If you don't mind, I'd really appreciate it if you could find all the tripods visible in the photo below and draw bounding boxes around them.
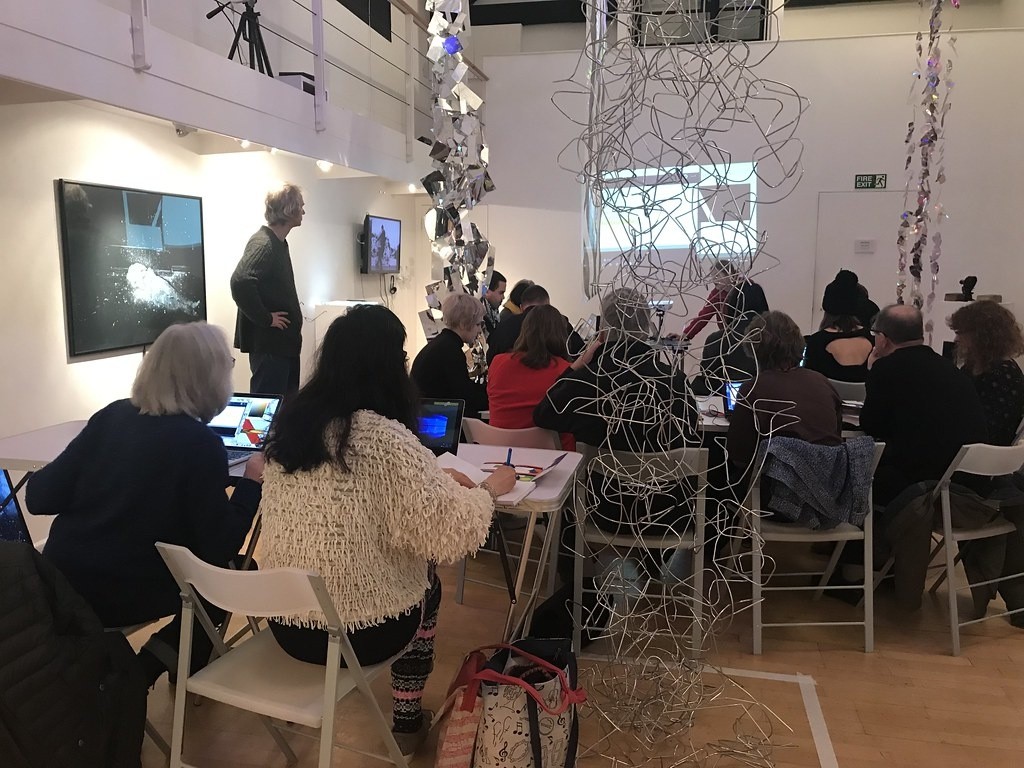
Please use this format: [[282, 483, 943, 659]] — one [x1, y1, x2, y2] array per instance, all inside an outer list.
[[206, 0, 275, 78]]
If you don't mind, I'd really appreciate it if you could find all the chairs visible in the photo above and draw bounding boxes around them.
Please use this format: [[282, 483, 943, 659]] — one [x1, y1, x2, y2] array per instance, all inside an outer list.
[[154, 540, 429, 768], [455, 416, 561, 605], [827, 376, 867, 402], [572, 443, 708, 663], [0, 541, 174, 768], [722, 438, 886, 656], [857, 438, 1024, 658]]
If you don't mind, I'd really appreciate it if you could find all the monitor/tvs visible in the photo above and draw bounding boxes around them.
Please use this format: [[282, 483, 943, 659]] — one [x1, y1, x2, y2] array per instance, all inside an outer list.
[[360, 215, 401, 274]]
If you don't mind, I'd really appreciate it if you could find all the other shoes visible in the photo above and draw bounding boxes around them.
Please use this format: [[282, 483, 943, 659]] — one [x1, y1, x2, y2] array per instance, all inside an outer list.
[[558, 554, 576, 588], [812, 572, 863, 607], [169, 640, 211, 686], [596, 554, 640, 613], [661, 547, 693, 598]]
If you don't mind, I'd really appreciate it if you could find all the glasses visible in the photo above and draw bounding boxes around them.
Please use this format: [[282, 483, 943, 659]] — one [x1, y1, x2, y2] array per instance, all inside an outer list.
[[472, 319, 485, 330], [870, 323, 889, 339]]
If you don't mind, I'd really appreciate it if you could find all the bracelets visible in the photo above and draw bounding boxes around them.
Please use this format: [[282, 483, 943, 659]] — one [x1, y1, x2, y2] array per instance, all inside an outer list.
[[479, 481, 496, 501]]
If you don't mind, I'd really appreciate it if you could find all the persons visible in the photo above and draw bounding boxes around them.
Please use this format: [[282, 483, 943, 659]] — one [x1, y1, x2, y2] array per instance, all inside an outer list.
[[230, 183, 305, 410], [26, 320, 266, 685], [375, 224, 386, 270], [258, 304, 518, 765], [410, 260, 1024, 608]]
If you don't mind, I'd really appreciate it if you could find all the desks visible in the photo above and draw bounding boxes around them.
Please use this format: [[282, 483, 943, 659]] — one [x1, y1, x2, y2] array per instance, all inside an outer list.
[[696, 396, 863, 440], [0, 420, 583, 644]]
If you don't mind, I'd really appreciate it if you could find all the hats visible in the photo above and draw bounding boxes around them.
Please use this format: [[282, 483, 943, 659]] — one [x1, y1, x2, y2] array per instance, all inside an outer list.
[[823, 269, 869, 315], [601, 288, 650, 331]]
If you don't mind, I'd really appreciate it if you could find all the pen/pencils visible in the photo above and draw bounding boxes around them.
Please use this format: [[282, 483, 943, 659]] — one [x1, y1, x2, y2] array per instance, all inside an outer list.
[[506, 448, 512, 466]]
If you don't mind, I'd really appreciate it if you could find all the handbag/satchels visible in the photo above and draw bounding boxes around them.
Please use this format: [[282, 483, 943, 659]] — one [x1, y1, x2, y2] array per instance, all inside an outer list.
[[433, 636, 590, 768], [529, 575, 613, 650]]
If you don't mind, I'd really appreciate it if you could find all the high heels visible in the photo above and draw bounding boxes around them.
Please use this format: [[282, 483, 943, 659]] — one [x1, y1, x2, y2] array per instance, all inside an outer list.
[[393, 707, 434, 756]]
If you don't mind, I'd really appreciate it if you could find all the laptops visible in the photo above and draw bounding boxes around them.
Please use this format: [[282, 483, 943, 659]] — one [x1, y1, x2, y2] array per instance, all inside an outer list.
[[205, 392, 284, 467], [722, 378, 744, 419], [413, 398, 465, 457]]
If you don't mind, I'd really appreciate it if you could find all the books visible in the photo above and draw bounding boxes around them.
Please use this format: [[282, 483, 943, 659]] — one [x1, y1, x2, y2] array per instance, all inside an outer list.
[[435, 452, 537, 508]]
